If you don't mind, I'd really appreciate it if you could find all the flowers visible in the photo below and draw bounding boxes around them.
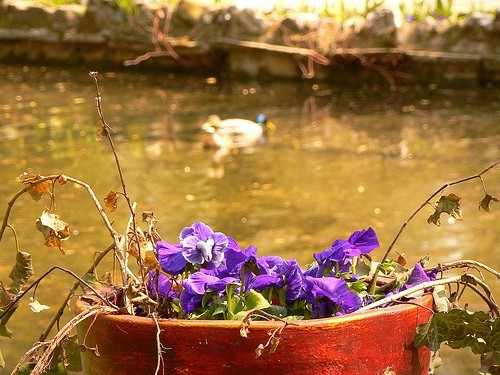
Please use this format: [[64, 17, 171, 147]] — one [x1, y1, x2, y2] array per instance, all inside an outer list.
[[0, 71, 500, 375]]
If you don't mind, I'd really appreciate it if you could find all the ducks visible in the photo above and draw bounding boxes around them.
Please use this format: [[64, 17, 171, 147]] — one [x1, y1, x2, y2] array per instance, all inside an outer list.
[[202, 112, 277, 145]]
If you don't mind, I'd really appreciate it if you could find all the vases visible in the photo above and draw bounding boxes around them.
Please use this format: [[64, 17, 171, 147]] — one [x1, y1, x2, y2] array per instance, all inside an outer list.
[[75, 273, 441, 375]]
